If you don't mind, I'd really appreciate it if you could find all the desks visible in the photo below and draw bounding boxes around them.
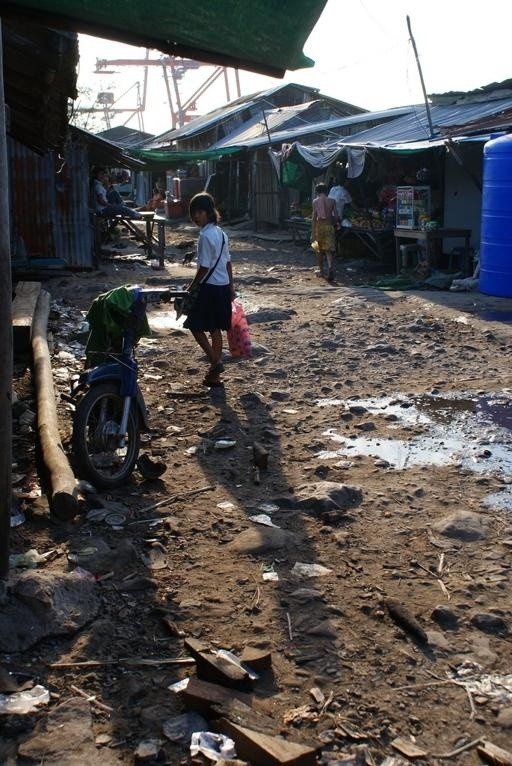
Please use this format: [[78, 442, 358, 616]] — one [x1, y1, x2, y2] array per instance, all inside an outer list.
[[92, 213, 167, 270], [285, 212, 392, 271], [393, 227, 474, 279]]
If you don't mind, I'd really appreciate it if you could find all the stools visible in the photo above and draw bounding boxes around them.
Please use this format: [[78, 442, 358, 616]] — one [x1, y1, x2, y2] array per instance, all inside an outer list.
[[398, 241, 426, 272], [444, 240, 479, 276]]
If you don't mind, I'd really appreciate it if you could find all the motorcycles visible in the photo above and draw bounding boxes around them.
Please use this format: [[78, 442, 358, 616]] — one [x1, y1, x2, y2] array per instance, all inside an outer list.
[[72, 282, 189, 492]]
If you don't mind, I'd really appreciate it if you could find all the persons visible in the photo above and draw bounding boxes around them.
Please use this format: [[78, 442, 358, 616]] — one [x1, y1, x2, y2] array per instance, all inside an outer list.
[[327, 179, 366, 243], [309, 183, 341, 280], [177, 192, 235, 388], [93, 171, 144, 221], [155, 177, 164, 195], [144, 188, 164, 206]]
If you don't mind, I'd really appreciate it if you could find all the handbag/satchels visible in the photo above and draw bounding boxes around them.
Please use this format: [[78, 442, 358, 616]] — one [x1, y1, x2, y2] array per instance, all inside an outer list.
[[174, 283, 199, 313]]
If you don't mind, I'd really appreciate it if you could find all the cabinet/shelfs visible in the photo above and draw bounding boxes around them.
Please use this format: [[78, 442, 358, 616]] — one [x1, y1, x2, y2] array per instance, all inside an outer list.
[[396, 184, 431, 229]]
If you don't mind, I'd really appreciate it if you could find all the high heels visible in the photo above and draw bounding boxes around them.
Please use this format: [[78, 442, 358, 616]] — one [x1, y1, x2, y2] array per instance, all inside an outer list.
[[204, 360, 224, 384]]
